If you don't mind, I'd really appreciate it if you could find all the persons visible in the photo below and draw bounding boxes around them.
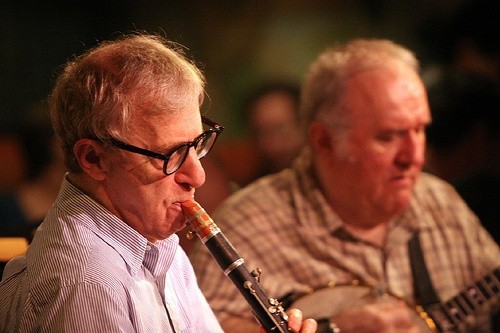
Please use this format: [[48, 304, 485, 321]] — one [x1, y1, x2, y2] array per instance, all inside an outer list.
[[240, 74, 308, 177], [0, 34, 320, 332], [186, 36, 500, 333], [0, 94, 69, 283]]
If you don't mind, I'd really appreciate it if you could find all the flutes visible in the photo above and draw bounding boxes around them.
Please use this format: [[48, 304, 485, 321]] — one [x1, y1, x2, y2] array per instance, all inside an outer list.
[[182, 198, 296, 333]]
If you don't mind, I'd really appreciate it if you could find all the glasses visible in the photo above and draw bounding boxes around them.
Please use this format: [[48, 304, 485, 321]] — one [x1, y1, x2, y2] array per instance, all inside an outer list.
[[88, 116, 225, 175]]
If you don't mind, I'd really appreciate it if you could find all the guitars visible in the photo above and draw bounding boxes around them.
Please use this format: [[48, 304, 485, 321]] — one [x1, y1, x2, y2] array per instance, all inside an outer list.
[[279, 264, 500, 333]]
